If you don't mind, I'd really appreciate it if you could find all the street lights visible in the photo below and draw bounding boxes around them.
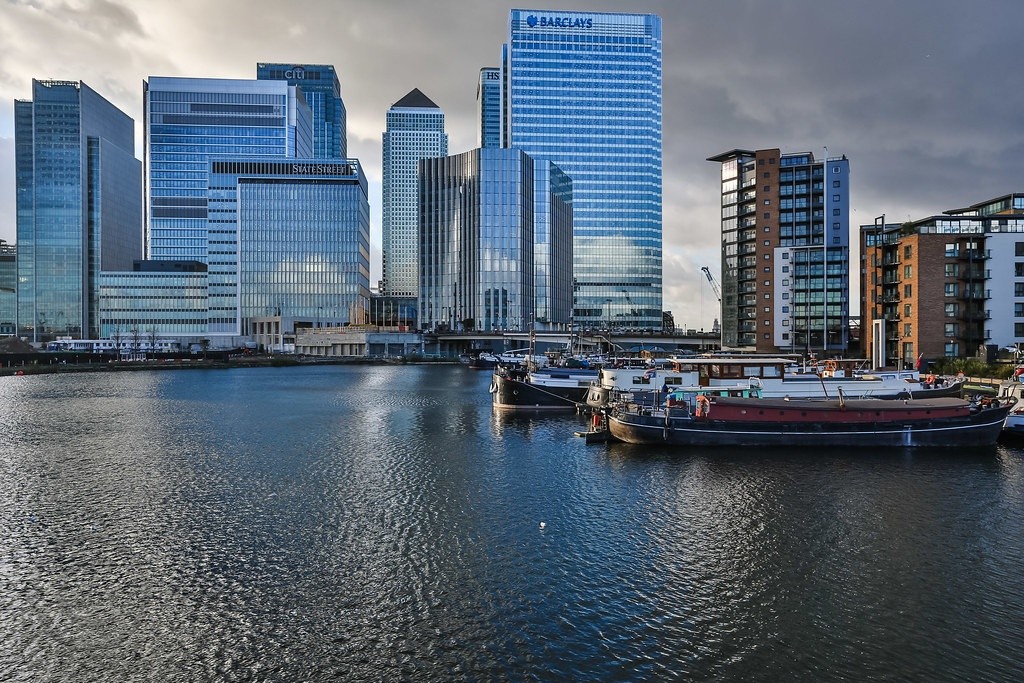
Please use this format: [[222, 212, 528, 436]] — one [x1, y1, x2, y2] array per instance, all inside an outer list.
[[606, 299, 614, 361]]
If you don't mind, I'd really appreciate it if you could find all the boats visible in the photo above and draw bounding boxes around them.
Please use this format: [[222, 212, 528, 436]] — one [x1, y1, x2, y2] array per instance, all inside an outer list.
[[466, 310, 1018, 452], [993, 368, 1024, 441]]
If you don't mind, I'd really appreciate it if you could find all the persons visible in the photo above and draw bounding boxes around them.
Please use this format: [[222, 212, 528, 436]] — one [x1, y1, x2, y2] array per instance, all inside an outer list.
[[895, 388, 914, 400]]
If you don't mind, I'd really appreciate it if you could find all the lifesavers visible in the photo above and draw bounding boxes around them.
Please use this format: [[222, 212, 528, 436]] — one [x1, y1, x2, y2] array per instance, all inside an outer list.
[[651, 359, 655, 363], [927, 374, 934, 384]]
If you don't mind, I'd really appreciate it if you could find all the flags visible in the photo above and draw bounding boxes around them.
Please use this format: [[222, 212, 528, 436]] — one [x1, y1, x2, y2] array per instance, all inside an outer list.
[[644, 368, 656, 379]]
